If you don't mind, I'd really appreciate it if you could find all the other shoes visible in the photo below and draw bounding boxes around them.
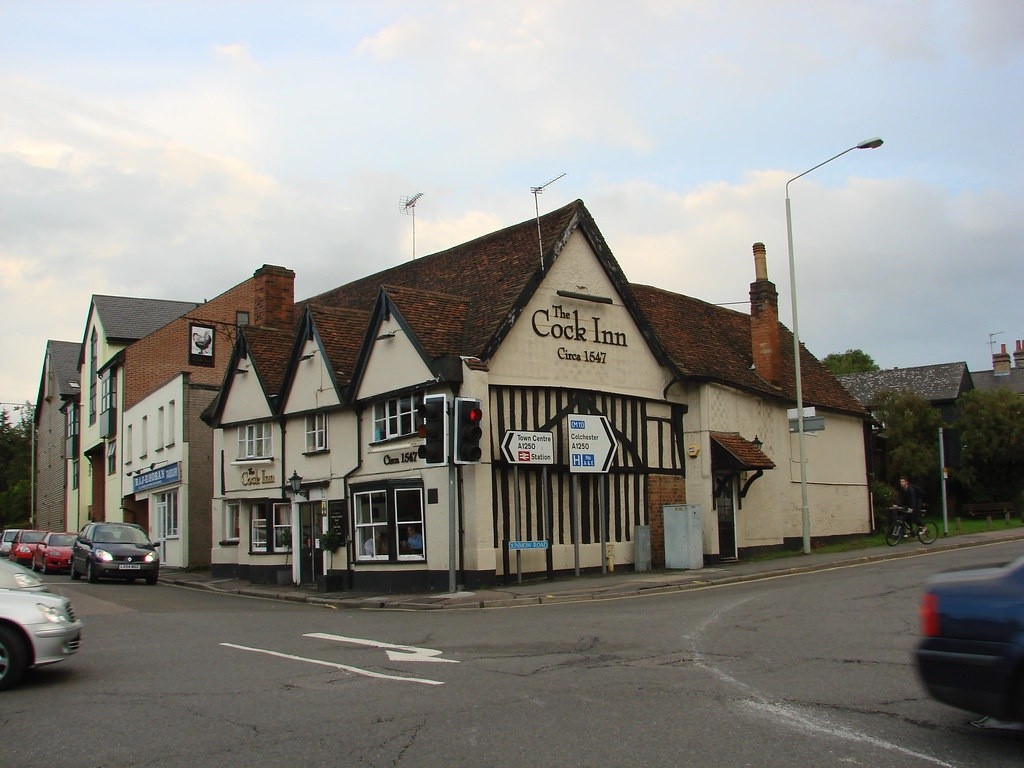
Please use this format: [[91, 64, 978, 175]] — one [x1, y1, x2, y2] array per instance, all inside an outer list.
[[918, 527, 928, 535], [903, 533, 911, 537]]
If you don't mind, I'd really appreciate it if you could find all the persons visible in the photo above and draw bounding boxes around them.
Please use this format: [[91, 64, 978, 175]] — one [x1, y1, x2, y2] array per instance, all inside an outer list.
[[406, 525, 422, 549], [893, 476, 928, 537], [364, 530, 386, 555]]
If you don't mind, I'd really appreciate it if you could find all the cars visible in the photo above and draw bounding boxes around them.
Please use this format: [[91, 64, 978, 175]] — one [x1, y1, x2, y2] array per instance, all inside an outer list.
[[0, 529, 20, 556], [9, 530, 49, 564], [32, 531, 81, 575], [909, 552, 1023, 725], [70, 522, 161, 585], [0, 588, 83, 692], [0, 556, 50, 593]]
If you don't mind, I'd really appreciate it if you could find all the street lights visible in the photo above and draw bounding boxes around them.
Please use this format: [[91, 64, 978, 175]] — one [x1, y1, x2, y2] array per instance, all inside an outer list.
[[785, 134, 885, 556]]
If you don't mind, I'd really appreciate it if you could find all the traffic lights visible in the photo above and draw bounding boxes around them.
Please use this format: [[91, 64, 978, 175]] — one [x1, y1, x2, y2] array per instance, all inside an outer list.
[[416, 393, 446, 465], [451, 397, 483, 465]]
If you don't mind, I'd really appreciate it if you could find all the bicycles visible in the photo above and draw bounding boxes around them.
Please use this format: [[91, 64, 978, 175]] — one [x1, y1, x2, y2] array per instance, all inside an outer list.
[[886, 503, 939, 547]]
